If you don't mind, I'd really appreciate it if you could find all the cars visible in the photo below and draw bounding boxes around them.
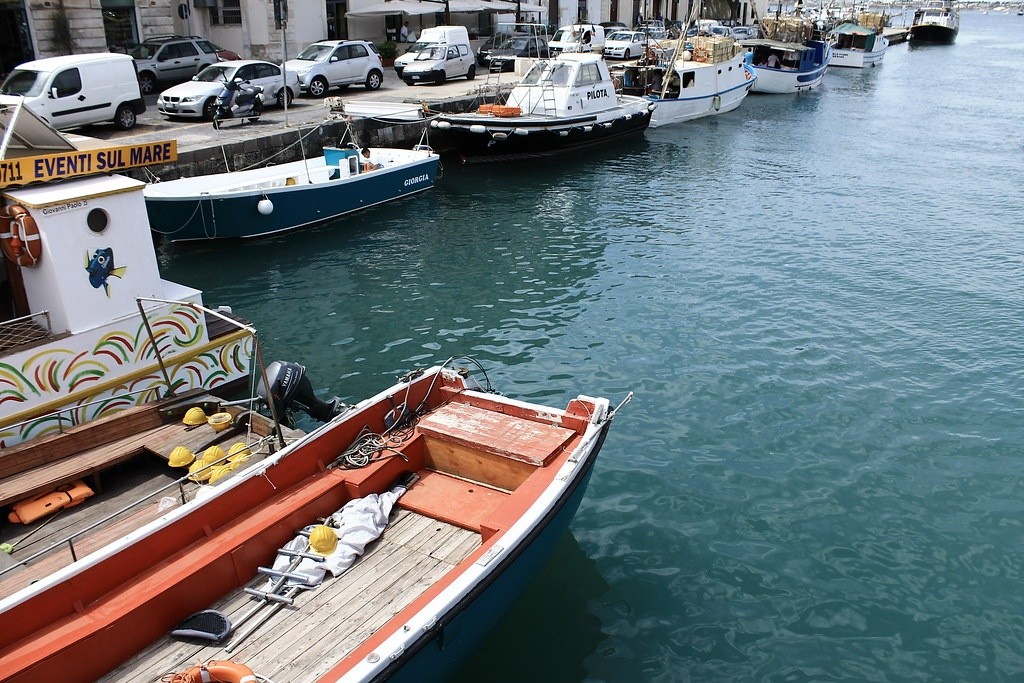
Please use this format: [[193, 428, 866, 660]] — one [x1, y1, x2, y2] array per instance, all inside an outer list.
[[156, 59, 300, 122], [476, 31, 534, 69], [484, 36, 549, 72], [573, 15, 759, 59], [211, 43, 242, 62], [401, 43, 477, 86]]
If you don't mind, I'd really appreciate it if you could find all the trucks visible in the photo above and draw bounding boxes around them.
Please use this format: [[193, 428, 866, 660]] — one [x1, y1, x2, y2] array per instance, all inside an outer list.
[[393, 26, 470, 80]]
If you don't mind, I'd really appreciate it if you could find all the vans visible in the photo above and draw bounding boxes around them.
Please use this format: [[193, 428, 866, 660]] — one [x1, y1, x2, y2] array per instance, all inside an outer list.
[[548, 24, 606, 54], [0, 53, 146, 134]]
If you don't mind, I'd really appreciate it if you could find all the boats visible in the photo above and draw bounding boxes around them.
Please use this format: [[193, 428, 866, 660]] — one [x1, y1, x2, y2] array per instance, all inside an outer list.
[[422, 51, 659, 168], [142, 95, 440, 249], [908, 0, 960, 41], [981, 2, 1024, 16], [740, 0, 834, 95], [0, 355, 614, 683], [825, 21, 890, 68], [0, 178, 353, 596], [771, 0, 902, 41], [609, 0, 759, 129]]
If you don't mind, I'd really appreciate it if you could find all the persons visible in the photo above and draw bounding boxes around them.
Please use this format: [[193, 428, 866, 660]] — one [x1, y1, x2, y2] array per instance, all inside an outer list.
[[361, 148, 381, 172], [647, 70, 662, 95], [614, 76, 621, 94], [624, 68, 634, 87], [519, 16, 526, 32], [530, 17, 537, 28], [744, 47, 754, 65], [401, 21, 409, 43], [767, 53, 780, 68]]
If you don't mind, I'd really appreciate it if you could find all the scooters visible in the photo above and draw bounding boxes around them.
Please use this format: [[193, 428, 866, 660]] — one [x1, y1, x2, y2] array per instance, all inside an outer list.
[[211, 68, 264, 129]]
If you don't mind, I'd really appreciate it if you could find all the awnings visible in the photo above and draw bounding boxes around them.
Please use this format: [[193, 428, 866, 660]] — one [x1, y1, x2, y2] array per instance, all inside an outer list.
[[344, 0, 547, 18]]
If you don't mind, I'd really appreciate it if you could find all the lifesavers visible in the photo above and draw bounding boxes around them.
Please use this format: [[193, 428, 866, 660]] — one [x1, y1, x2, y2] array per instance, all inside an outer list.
[[693, 46, 708, 62], [168, 659, 261, 683], [479, 103, 497, 114], [491, 105, 520, 117], [0, 204, 41, 268]]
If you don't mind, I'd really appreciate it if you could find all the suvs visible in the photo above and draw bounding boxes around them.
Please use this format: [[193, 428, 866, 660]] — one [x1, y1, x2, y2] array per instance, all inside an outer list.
[[280, 38, 384, 99], [124, 35, 220, 97]]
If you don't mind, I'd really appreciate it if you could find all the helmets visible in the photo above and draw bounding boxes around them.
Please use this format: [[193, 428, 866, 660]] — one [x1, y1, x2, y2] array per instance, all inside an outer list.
[[226, 443, 252, 461], [230, 454, 249, 472], [169, 445, 195, 466], [256, 92, 266, 105], [206, 412, 232, 431], [188, 459, 213, 480], [202, 445, 225, 469], [210, 467, 232, 485], [309, 525, 337, 556], [182, 406, 209, 425]]
